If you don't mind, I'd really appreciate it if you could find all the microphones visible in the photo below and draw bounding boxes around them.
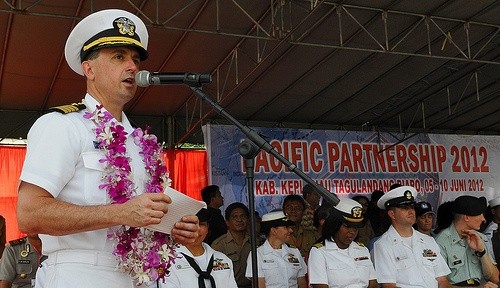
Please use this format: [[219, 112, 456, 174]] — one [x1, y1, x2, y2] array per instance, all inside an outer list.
[[135, 70, 212, 87]]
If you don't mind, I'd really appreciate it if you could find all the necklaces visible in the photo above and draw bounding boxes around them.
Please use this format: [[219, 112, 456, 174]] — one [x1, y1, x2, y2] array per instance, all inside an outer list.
[[80, 101, 184, 288]]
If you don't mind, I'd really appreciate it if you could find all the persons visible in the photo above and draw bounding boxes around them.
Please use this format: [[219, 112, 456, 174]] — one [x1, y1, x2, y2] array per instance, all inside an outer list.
[[245, 211, 308, 288], [16, 9, 200, 288], [370, 186, 453, 288], [248, 184, 500, 269], [211, 202, 263, 288], [160, 207, 238, 288], [435, 197, 500, 288], [307, 198, 377, 288], [196, 184, 228, 246], [0, 233, 43, 288]]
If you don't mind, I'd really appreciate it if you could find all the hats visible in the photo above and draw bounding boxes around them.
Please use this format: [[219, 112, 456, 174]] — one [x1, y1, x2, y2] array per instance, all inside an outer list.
[[262, 211, 296, 227], [488, 197, 500, 207], [452, 195, 485, 215], [65, 9, 149, 76], [415, 201, 436, 217], [195, 201, 215, 222], [328, 198, 367, 227], [377, 186, 418, 210]]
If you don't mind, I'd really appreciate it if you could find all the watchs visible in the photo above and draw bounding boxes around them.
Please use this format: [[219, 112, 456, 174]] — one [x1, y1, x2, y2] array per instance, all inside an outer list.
[[474, 249, 486, 258]]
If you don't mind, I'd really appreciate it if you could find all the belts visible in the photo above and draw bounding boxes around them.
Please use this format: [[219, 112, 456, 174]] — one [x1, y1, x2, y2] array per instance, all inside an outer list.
[[454, 279, 480, 287]]
